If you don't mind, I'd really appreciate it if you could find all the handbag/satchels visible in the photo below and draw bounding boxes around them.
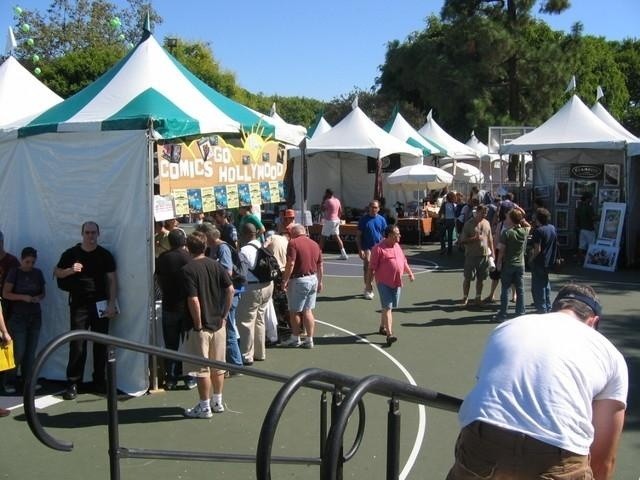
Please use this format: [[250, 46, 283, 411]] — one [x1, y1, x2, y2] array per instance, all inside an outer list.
[[0, 339, 16, 371], [177, 308, 193, 331], [56, 258, 72, 291], [216, 243, 242, 280], [481, 246, 488, 256]]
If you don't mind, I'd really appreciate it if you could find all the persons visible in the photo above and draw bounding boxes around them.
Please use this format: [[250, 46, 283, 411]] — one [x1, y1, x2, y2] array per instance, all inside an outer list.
[[377, 196, 398, 236], [51, 222, 117, 399], [366, 225, 415, 345], [424, 188, 557, 322], [447, 283, 629, 478], [354, 200, 387, 300], [3, 247, 46, 396], [0, 232, 21, 395], [158, 203, 322, 420], [318, 189, 349, 260], [0, 301, 12, 418]]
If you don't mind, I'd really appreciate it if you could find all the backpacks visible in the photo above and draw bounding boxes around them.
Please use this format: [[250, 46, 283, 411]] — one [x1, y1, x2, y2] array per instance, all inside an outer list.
[[245, 243, 281, 282]]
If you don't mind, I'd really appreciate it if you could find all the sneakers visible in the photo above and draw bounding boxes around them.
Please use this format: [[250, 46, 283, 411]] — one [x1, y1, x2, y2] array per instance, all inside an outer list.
[[0, 409, 10, 417], [364, 291, 374, 300], [336, 256, 348, 260], [531, 303, 550, 313], [185, 401, 224, 418], [463, 297, 517, 323], [265, 339, 314, 348], [166, 379, 197, 390], [380, 327, 397, 344], [440, 247, 446, 255]]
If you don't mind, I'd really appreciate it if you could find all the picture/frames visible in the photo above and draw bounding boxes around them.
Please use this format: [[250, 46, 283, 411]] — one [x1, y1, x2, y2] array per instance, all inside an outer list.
[[533, 163, 627, 271]]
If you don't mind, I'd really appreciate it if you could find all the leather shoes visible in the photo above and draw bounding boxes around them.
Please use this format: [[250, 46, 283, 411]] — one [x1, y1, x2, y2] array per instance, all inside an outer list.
[[63, 384, 77, 399], [94, 384, 108, 393]]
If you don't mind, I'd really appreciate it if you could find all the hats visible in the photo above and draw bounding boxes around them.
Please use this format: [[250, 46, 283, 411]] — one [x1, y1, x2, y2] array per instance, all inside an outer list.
[[284, 209, 295, 217]]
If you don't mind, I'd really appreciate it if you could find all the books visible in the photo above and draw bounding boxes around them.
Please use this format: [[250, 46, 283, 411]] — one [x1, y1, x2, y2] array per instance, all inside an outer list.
[[96, 299, 120, 318]]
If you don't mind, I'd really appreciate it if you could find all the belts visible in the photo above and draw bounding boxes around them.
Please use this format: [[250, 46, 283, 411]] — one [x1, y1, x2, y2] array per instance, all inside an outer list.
[[291, 272, 314, 278], [248, 281, 261, 284]]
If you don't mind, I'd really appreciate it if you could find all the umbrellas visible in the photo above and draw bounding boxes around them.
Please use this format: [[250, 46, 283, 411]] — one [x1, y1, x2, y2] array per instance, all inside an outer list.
[[387, 163, 453, 246], [440, 162, 484, 191]]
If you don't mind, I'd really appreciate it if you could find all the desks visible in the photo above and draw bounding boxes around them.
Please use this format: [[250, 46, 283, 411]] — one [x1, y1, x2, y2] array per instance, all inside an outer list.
[[153, 301, 167, 387], [308, 206, 444, 250]]
